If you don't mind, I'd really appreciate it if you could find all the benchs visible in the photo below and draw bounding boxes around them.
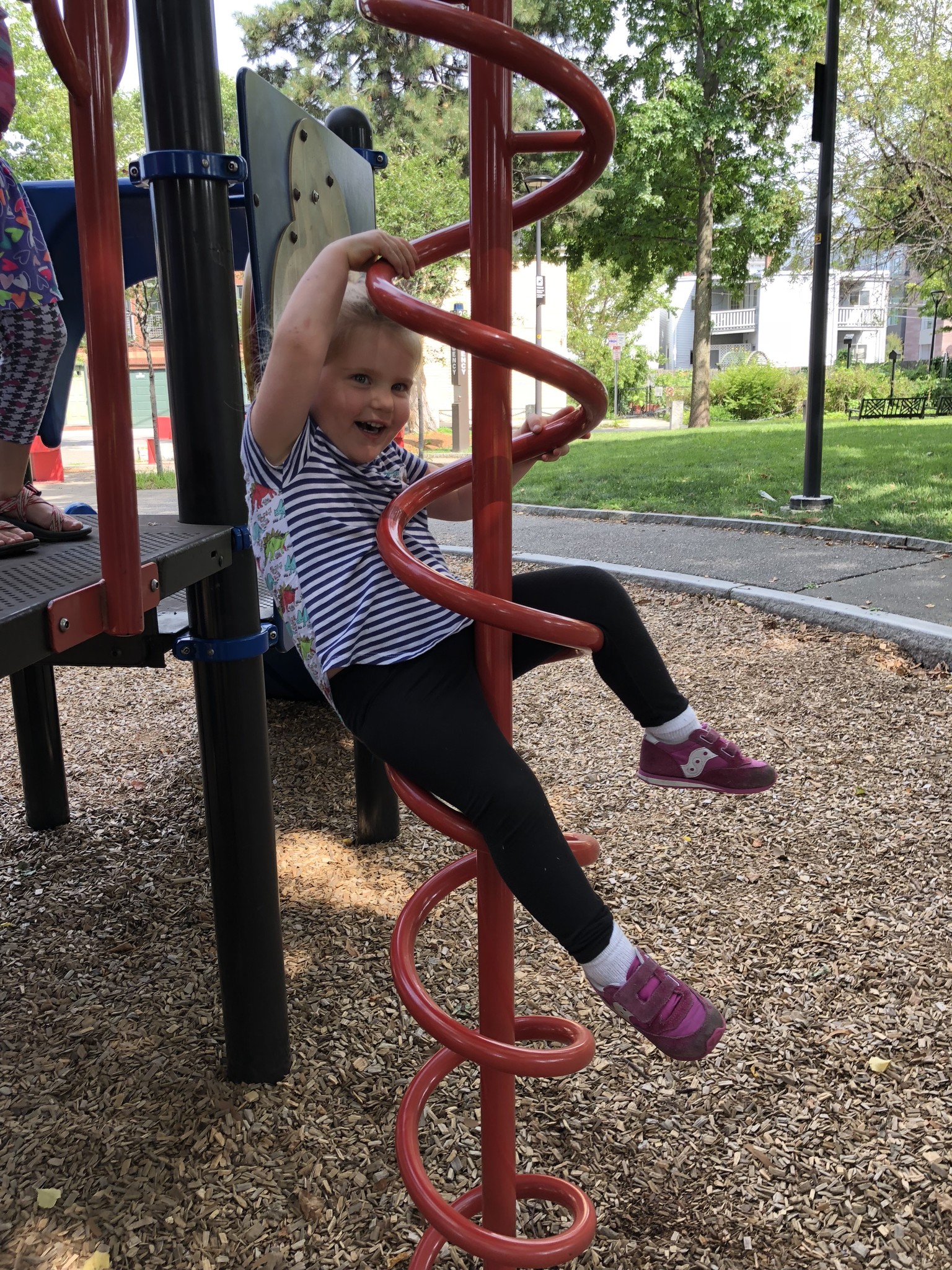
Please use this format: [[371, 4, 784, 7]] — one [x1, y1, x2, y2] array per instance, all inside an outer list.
[[922, 396, 952, 418], [848, 397, 927, 422]]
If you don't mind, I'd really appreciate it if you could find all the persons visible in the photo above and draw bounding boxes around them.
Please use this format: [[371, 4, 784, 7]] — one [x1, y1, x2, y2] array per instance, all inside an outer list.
[[238, 232, 778, 1065], [0, 13, 92, 561]]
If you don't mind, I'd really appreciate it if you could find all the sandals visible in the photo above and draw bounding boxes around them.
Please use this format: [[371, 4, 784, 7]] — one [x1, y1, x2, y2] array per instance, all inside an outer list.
[[0, 486, 93, 541], [0, 521, 40, 555]]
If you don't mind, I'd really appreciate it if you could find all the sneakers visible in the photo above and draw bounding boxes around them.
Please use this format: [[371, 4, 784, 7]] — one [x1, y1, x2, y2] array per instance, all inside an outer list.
[[585, 946, 726, 1062], [637, 723, 777, 794]]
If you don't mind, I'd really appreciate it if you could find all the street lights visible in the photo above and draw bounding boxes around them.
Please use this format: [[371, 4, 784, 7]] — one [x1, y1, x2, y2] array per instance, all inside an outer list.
[[927, 290, 946, 376], [523, 174, 556, 416]]
